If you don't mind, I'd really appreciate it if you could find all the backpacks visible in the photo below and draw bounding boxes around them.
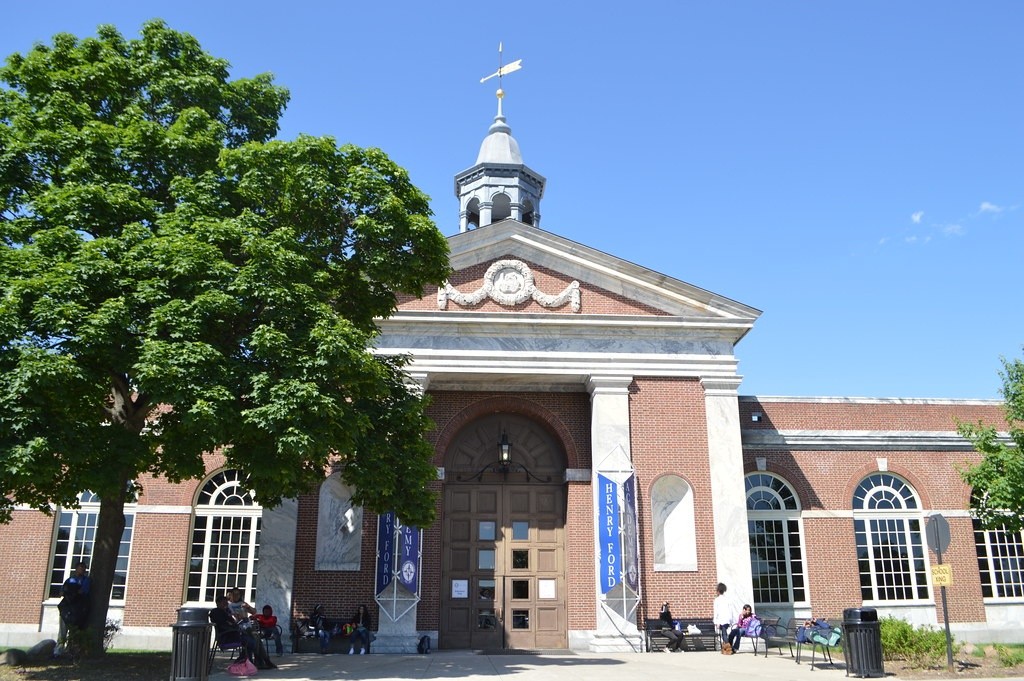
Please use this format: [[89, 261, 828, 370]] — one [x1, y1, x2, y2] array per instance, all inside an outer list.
[[744, 618, 762, 637]]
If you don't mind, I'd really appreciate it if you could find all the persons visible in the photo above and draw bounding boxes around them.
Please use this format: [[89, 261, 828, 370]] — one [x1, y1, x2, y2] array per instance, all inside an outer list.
[[210, 596, 256, 664], [249, 605, 283, 657], [660, 602, 685, 654], [728, 604, 757, 653], [713, 583, 734, 655], [348, 605, 371, 655], [305, 604, 331, 654], [226, 588, 277, 670], [53, 562, 91, 659], [798, 617, 842, 647]]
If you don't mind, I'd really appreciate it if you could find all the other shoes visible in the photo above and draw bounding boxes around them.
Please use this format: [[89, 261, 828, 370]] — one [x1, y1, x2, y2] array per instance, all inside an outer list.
[[829, 628, 841, 646], [277, 653, 282, 657], [360, 648, 365, 655], [349, 648, 354, 654], [54, 641, 63, 657], [675, 648, 684, 653], [322, 649, 328, 653], [663, 647, 670, 653], [722, 643, 733, 654], [810, 631, 828, 645], [264, 658, 277, 669], [257, 661, 270, 670]]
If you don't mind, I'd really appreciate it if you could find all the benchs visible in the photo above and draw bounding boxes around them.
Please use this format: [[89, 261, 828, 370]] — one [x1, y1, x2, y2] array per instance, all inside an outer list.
[[208, 614, 283, 674], [731, 616, 782, 656], [643, 617, 722, 652], [797, 618, 850, 677], [765, 618, 825, 662], [290, 618, 370, 654]]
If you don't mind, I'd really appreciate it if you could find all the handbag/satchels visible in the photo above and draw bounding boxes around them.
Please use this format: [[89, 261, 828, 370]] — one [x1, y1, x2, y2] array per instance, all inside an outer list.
[[228, 657, 257, 675], [687, 625, 701, 635], [673, 620, 680, 632]]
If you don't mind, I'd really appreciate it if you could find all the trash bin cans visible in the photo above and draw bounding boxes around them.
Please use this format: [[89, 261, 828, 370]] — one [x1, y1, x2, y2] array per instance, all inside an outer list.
[[841, 607, 886, 678], [169, 608, 216, 681]]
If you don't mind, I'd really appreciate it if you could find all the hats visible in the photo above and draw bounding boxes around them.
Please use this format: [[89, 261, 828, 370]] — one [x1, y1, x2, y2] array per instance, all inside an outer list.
[[216, 595, 226, 603], [314, 604, 324, 611]]
